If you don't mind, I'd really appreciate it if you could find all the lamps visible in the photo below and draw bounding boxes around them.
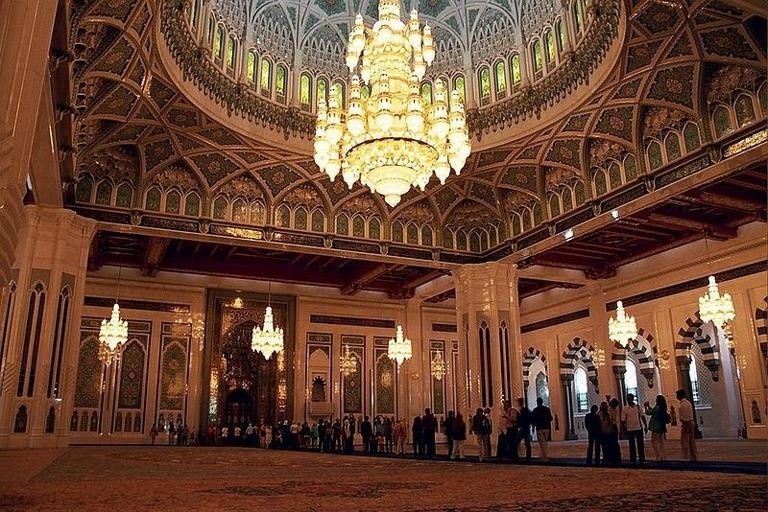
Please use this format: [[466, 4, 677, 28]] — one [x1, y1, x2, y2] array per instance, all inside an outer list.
[[251, 253, 285, 362], [608, 237, 638, 349], [689, 204, 736, 330], [98, 236, 129, 352], [388, 281, 413, 365], [312, 0, 473, 208]]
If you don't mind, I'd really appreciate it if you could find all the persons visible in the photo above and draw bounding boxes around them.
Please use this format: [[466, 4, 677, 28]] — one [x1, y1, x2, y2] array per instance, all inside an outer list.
[[413, 399, 554, 464], [621, 393, 648, 462], [676, 389, 698, 461], [643, 395, 666, 462], [169, 423, 216, 446], [245, 423, 273, 448], [585, 398, 622, 465], [149, 424, 159, 445], [276, 415, 354, 455], [222, 423, 241, 446], [360, 415, 410, 455]]
